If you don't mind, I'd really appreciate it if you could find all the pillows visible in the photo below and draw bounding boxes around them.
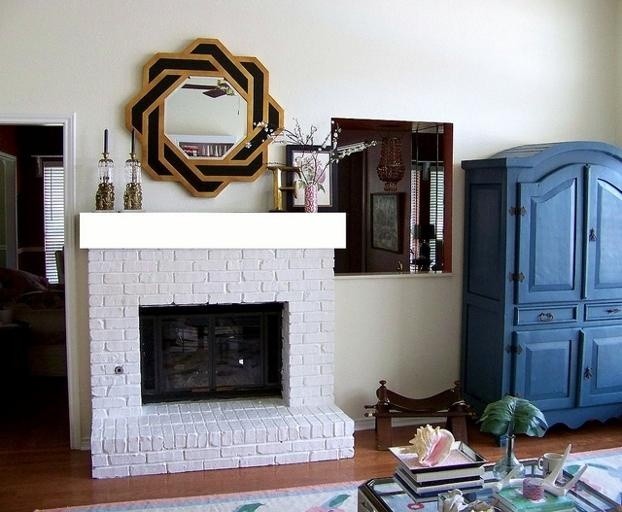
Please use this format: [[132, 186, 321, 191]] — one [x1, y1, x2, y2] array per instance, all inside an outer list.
[[1, 265, 49, 301]]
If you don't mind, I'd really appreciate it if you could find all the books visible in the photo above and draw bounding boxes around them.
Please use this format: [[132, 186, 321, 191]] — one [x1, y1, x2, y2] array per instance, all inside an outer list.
[[392, 467, 576, 512]]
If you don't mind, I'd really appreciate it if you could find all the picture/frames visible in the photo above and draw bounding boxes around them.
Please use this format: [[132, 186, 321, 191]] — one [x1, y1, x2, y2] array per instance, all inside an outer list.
[[369, 192, 402, 253], [287, 145, 337, 213]]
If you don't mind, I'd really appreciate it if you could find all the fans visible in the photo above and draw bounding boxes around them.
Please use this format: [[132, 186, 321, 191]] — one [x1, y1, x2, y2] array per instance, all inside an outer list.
[[182, 78, 242, 115]]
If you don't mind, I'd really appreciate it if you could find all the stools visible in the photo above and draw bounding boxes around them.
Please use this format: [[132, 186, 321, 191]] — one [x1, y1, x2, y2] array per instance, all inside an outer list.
[[365, 381, 474, 442]]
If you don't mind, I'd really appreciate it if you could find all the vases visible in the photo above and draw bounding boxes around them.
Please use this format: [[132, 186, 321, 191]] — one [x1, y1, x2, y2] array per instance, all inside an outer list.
[[304, 184, 318, 213]]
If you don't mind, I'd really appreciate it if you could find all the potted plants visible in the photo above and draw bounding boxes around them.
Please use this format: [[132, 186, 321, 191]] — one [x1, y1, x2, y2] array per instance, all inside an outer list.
[[475, 393, 548, 478]]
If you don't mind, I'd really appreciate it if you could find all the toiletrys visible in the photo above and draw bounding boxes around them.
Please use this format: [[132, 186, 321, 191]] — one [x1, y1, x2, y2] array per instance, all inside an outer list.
[[198, 145, 226, 157]]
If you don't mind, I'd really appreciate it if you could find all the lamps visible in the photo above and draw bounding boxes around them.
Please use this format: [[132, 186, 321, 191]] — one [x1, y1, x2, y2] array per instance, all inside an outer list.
[[414, 225, 433, 259]]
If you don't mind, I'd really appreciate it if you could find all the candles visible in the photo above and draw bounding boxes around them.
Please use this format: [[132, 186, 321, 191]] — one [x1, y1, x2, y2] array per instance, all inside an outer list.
[[132, 126, 136, 153], [103, 129, 109, 152]]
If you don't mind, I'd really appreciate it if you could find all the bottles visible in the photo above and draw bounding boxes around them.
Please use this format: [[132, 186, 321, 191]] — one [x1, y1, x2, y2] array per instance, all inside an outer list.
[[94, 152, 117, 213], [122, 152, 144, 210], [492, 435, 526, 481]]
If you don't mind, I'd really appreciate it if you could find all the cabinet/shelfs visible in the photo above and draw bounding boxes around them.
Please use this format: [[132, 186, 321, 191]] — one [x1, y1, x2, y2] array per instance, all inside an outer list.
[[460, 141, 621, 428]]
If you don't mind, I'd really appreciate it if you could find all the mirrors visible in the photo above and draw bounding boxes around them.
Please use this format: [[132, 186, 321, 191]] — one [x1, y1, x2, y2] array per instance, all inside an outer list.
[[126, 38, 286, 199]]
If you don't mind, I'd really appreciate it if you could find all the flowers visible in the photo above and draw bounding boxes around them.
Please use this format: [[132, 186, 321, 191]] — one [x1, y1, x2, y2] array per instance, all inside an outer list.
[[245, 113, 378, 191]]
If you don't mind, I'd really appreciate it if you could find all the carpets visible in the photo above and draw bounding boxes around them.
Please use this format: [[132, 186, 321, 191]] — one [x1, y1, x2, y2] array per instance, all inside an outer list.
[[34, 446, 621, 512]]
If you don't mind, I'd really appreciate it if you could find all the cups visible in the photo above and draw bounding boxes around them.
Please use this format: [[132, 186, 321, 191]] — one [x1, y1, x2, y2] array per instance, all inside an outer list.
[[537, 452, 564, 480]]
